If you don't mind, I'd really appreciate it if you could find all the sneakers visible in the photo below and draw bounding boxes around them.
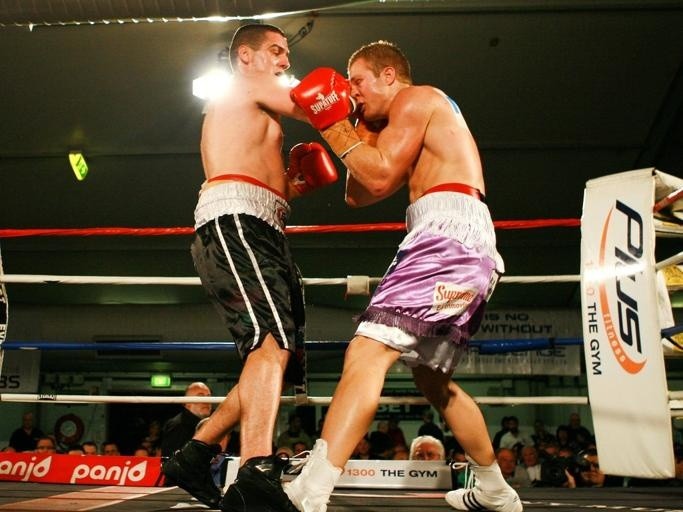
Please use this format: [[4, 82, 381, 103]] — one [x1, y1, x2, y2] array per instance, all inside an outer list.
[[161, 436, 222, 510], [221, 452, 303, 511], [443, 453, 523, 512]]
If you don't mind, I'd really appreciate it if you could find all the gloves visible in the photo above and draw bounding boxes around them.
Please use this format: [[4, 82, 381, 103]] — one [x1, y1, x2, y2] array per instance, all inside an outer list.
[[283, 139, 338, 198], [288, 65, 352, 133]]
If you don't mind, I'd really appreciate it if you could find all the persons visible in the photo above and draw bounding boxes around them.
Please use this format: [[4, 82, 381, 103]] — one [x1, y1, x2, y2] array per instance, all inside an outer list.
[[346, 408, 683, 490], [0, 409, 164, 458], [279, 39, 523, 512], [161, 23, 359, 512], [161, 380, 331, 485]]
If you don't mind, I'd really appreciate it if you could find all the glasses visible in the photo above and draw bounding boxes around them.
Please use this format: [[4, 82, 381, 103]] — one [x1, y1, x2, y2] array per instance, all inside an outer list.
[[35, 445, 56, 450]]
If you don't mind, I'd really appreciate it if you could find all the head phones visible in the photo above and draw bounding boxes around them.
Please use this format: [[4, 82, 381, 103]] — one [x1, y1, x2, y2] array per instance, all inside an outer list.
[[574, 448, 596, 479]]
